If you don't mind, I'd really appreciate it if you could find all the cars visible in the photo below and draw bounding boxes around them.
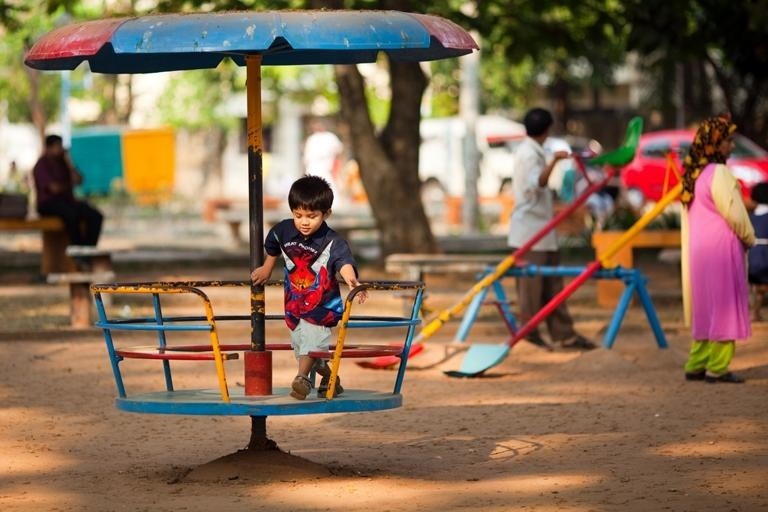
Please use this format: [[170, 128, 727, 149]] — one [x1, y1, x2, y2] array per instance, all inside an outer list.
[[415, 112, 547, 219], [619, 123, 768, 217]]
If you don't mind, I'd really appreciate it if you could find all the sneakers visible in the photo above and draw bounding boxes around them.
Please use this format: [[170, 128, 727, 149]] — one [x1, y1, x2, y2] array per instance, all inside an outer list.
[[290, 375, 312, 400], [317, 377, 343, 398], [525, 335, 595, 349]]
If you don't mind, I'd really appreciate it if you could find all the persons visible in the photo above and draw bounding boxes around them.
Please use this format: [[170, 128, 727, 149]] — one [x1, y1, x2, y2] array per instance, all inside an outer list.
[[6, 161, 21, 189], [33, 135, 103, 271], [302, 121, 345, 215], [506, 108, 597, 352], [748, 178, 768, 285], [649, 107, 665, 132], [683, 111, 757, 383], [250, 175, 369, 400]]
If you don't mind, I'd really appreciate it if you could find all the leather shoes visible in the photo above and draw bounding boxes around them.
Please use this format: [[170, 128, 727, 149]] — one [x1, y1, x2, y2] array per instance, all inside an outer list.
[[686, 371, 744, 382]]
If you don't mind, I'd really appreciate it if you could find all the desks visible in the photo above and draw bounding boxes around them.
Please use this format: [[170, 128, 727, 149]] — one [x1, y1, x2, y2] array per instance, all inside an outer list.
[[0, 216, 74, 276]]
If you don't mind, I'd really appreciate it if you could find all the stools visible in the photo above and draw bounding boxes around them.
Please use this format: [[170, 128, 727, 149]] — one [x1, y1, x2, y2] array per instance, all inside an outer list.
[[45, 241, 131, 331]]
